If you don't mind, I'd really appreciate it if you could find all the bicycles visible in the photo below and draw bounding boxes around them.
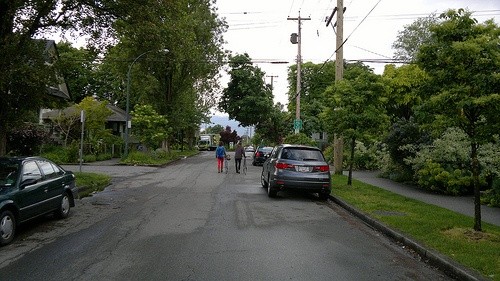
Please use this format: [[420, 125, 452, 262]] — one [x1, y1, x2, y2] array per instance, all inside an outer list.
[[242, 155, 250, 174], [224, 154, 231, 175]]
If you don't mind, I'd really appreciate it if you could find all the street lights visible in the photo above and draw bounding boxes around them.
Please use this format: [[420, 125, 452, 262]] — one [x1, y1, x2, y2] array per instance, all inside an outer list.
[[121, 48, 169, 163]]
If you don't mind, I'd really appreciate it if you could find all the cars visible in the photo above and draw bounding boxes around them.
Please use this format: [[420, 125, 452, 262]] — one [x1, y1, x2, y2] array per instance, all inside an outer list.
[[245, 145, 255, 152], [260, 143, 334, 203], [252, 145, 274, 167], [0, 155, 78, 248]]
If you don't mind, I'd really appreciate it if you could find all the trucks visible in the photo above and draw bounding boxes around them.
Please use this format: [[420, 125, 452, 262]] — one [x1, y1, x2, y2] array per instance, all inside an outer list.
[[197, 135, 218, 151]]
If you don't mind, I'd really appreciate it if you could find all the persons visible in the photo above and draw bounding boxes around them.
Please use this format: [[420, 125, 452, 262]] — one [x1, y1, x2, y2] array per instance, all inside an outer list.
[[215, 141, 227, 173], [234, 142, 246, 175]]
